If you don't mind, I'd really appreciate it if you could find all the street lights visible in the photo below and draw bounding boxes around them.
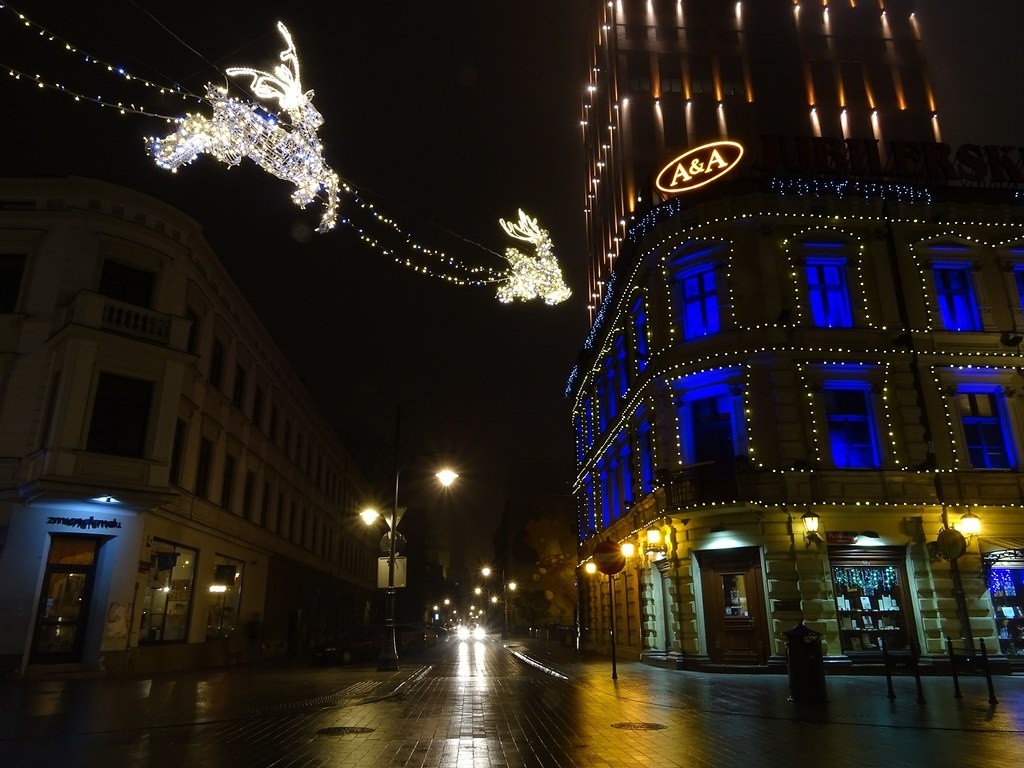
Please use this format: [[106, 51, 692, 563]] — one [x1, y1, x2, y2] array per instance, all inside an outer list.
[[482, 566, 509, 640], [379, 464, 460, 672]]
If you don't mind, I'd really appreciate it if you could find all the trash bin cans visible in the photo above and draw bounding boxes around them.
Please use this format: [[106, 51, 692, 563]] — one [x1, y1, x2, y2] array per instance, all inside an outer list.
[[781, 623, 827, 693]]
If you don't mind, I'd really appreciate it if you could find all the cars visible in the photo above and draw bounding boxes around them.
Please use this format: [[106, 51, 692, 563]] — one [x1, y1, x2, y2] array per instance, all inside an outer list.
[[311, 620, 447, 667]]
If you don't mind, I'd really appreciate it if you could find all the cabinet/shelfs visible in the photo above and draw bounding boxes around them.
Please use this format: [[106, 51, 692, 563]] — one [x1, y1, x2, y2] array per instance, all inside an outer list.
[[992, 596, 1024, 654], [839, 608, 904, 635]]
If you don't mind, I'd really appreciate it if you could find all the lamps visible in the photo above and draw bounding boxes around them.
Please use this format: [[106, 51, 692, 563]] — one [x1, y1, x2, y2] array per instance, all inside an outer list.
[[711, 522, 725, 533], [648, 526, 660, 544], [801, 508, 820, 546], [958, 506, 982, 539]]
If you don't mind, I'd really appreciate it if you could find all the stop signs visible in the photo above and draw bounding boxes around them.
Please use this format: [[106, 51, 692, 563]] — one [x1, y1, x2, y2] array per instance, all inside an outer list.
[[594, 542, 626, 575]]
